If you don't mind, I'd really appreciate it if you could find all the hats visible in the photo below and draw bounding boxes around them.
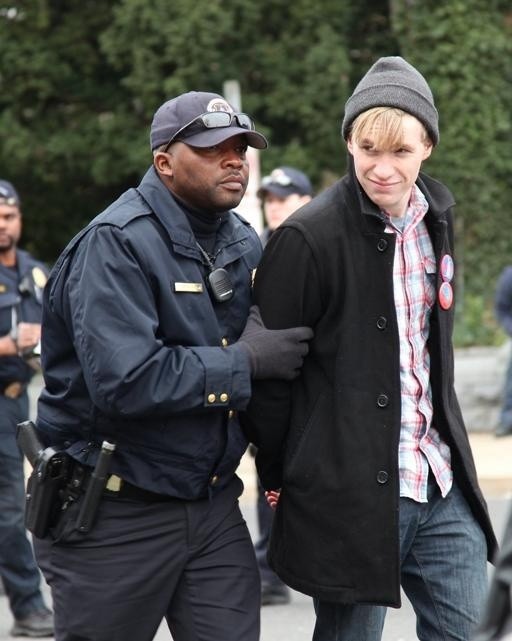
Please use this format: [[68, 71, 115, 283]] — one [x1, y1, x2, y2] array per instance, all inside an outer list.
[[342, 57, 439, 148], [0, 180, 20, 206], [257, 166, 314, 196], [149, 91, 268, 150]]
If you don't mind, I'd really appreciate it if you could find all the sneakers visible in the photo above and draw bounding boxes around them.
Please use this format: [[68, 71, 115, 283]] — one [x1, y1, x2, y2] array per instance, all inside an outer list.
[[12, 611, 53, 637], [260, 580, 290, 604]]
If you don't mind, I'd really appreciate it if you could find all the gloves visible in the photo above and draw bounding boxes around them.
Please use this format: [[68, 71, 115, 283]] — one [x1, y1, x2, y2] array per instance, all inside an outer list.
[[238, 304, 315, 383]]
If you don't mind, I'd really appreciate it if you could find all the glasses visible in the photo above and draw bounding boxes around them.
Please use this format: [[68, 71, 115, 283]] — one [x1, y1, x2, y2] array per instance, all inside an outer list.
[[163, 110, 256, 153], [262, 175, 307, 194]]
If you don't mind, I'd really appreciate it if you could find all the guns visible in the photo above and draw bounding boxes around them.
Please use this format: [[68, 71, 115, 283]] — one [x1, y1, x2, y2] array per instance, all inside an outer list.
[[15, 422, 68, 539]]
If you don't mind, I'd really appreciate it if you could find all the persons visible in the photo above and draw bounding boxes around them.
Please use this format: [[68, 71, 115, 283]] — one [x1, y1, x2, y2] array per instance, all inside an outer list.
[[231, 165, 319, 607], [486, 262, 510, 440], [30, 89, 317, 641], [248, 51, 504, 641], [0, 178, 61, 641]]
